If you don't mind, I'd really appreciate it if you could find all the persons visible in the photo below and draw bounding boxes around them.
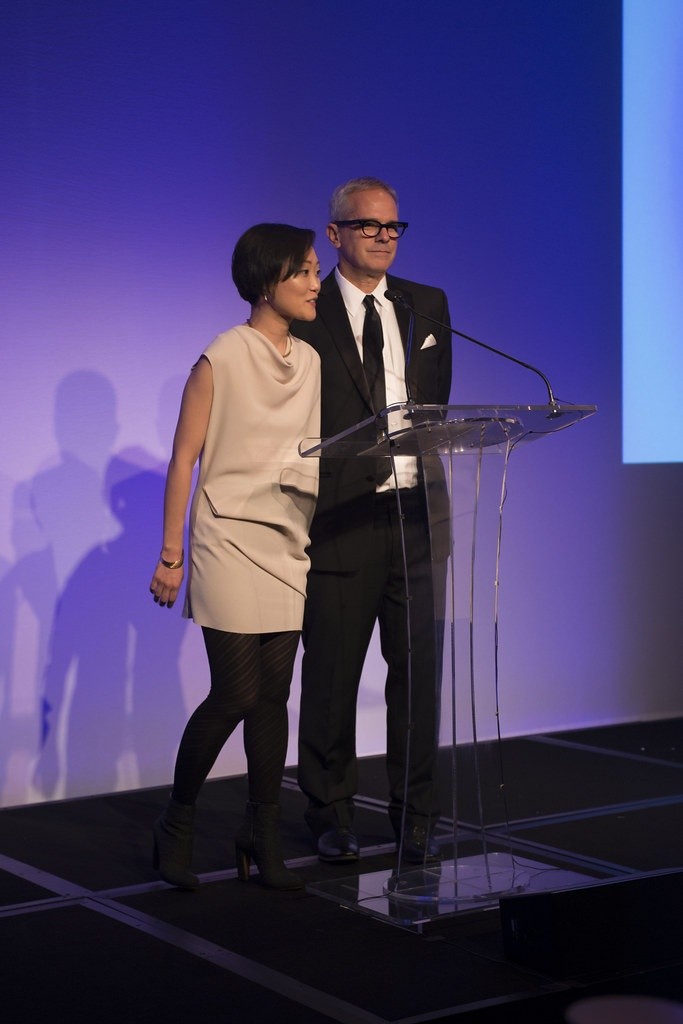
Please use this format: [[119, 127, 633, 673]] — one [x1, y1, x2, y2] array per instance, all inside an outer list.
[[149, 223, 323, 891], [290, 176, 453, 865]]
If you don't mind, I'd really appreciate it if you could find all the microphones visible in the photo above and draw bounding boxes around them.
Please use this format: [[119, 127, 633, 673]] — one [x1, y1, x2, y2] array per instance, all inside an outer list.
[[383, 290, 564, 420], [374, 417, 396, 447]]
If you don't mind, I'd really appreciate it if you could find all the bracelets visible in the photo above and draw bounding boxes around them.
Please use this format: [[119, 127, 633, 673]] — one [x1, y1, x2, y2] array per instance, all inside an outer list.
[[160, 549, 184, 568]]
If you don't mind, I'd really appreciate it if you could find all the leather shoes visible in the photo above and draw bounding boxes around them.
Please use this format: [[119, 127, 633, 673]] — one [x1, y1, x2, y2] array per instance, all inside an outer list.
[[396, 825, 444, 863], [317, 826, 361, 861]]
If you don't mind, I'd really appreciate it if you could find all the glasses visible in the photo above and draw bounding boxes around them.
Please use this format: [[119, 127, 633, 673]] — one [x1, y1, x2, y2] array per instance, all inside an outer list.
[[333, 219, 408, 239]]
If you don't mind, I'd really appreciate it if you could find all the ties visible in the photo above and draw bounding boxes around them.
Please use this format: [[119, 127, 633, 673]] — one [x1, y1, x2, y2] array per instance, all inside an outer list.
[[359, 294, 393, 487]]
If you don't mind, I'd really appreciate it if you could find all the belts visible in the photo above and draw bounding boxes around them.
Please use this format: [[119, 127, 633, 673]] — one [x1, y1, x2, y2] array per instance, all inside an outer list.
[[375, 482, 426, 513]]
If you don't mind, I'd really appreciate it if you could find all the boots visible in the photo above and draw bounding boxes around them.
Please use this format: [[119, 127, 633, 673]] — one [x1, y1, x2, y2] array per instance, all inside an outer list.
[[152, 792, 200, 890], [234, 800, 305, 892]]
